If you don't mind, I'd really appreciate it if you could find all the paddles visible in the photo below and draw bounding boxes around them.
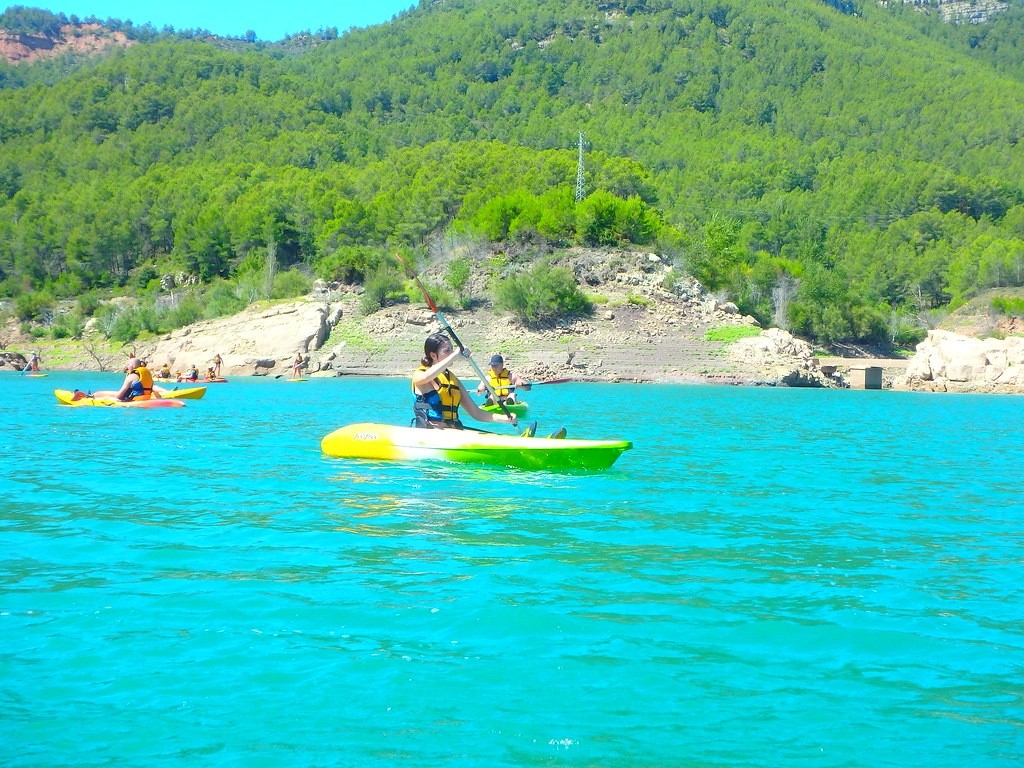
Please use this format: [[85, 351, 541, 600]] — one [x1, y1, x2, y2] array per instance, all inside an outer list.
[[467, 377, 573, 394], [121, 344, 162, 399], [19, 361, 31, 376], [395, 252, 523, 436], [274, 360, 306, 379], [71, 391, 93, 402]]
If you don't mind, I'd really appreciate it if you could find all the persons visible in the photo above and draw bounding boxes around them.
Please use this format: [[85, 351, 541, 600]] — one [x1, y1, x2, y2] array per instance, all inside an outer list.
[[292, 353, 302, 378], [477, 354, 531, 406], [0, 354, 25, 370], [184, 365, 198, 378], [204, 354, 223, 380], [30, 351, 40, 371], [114, 358, 153, 402], [158, 363, 170, 378], [413, 334, 567, 438]]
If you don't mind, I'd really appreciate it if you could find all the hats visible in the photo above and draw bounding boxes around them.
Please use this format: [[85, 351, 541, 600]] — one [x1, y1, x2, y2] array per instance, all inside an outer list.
[[488, 354, 504, 366]]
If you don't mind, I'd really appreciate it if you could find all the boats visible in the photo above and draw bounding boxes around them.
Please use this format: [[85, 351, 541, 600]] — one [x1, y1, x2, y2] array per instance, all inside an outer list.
[[320, 422, 632, 477], [477, 401, 528, 419], [287, 379, 308, 382], [149, 387, 206, 399], [54, 389, 186, 408], [26, 373, 49, 377], [152, 377, 228, 383]]
